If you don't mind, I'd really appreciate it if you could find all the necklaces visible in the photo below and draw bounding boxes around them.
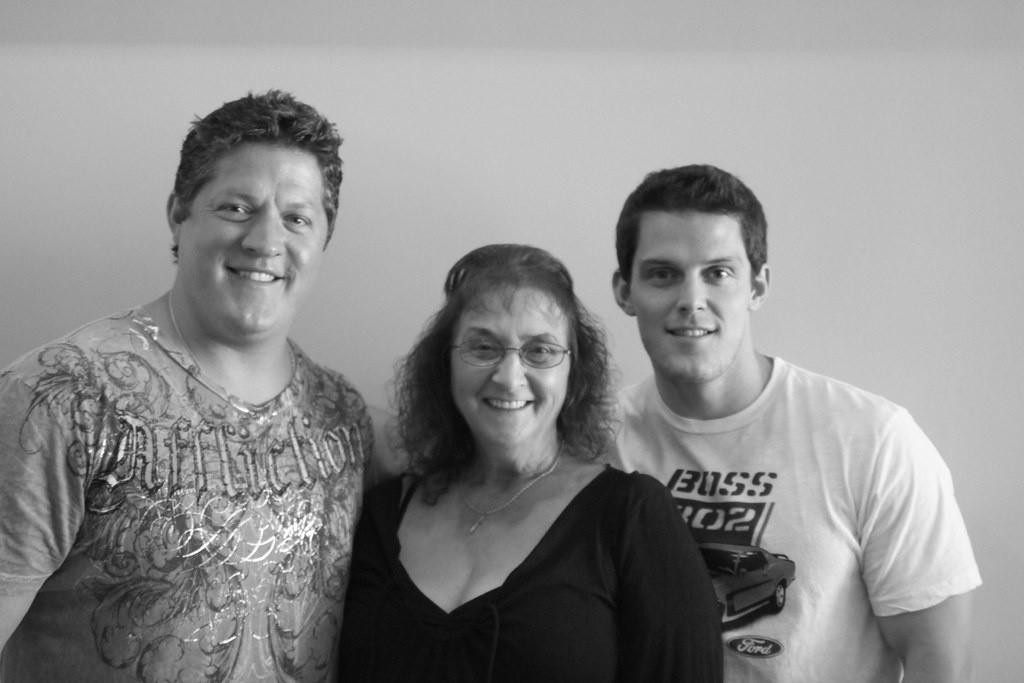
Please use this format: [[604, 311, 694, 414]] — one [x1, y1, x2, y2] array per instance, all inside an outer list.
[[170, 291, 295, 374], [459, 441, 565, 532]]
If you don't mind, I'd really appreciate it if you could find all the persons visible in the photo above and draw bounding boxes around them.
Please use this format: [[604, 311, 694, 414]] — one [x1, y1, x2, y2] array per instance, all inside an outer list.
[[592, 165, 983, 682], [1, 89, 417, 683], [335, 244, 725, 682]]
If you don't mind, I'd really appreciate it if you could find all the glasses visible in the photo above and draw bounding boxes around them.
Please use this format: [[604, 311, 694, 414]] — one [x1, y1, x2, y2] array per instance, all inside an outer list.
[[451, 339, 572, 369]]
